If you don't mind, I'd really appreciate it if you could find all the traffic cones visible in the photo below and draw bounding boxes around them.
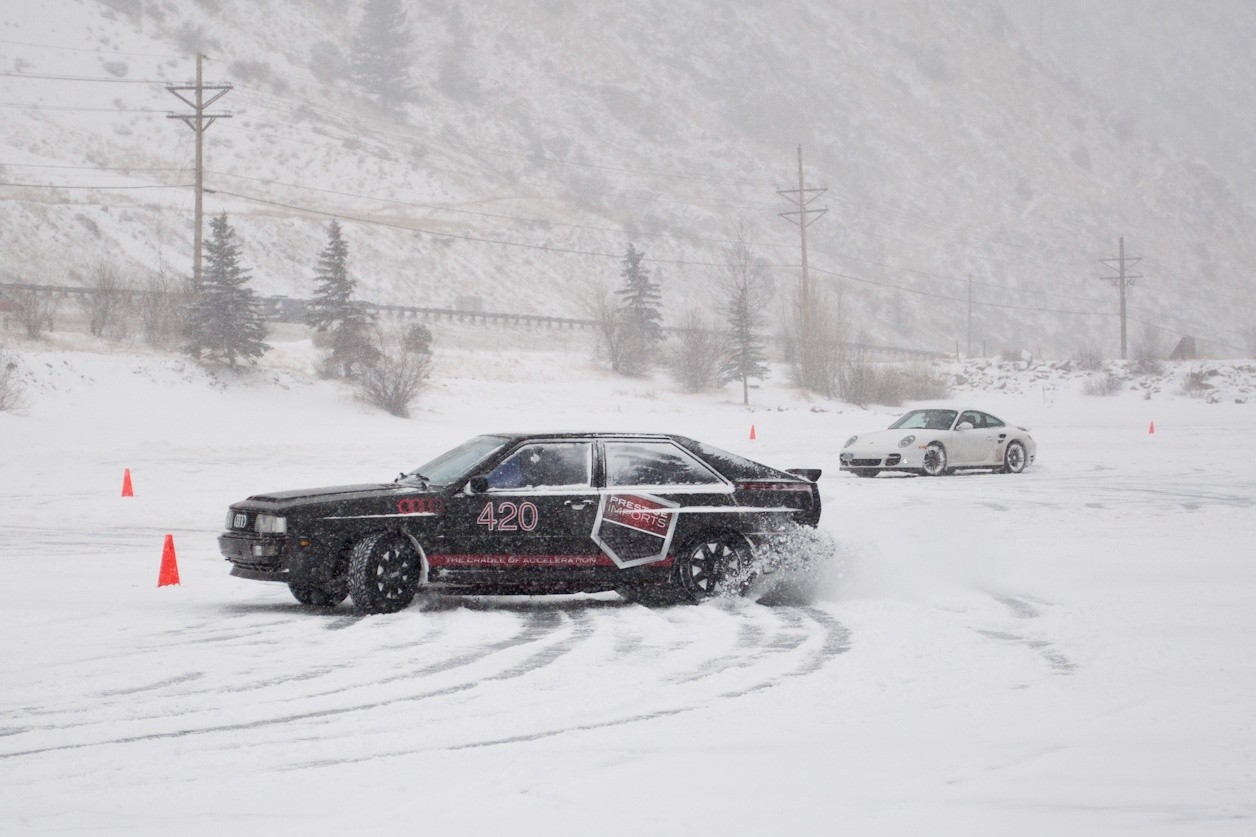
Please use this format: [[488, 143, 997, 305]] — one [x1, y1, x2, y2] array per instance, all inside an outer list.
[[123, 469, 134, 497], [1149, 422, 1154, 434], [158, 535, 179, 587], [750, 425, 755, 441]]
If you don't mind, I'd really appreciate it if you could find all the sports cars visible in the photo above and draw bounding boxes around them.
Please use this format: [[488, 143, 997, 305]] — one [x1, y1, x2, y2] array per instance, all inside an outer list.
[[840, 409, 1036, 478], [223, 432, 820, 613]]
